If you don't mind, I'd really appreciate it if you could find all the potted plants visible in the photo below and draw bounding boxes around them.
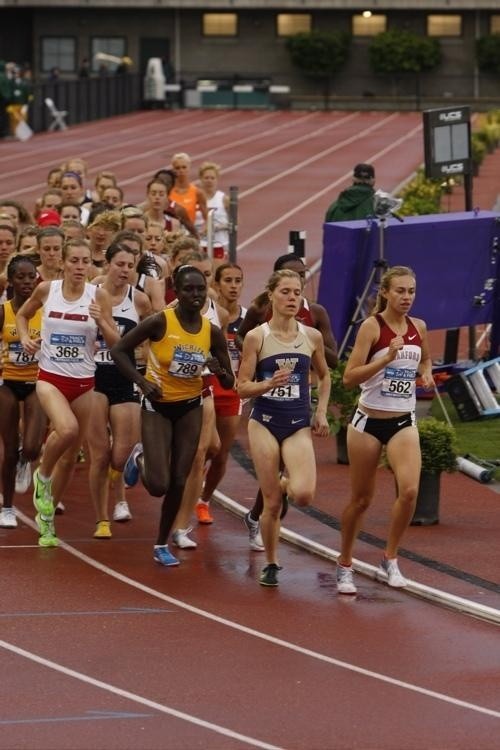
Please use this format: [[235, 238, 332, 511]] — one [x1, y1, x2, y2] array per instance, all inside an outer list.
[[387, 417, 457, 529], [305, 357, 358, 464]]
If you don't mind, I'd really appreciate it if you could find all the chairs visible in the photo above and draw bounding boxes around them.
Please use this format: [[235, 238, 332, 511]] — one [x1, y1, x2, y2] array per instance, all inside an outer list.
[[42, 98, 69, 132]]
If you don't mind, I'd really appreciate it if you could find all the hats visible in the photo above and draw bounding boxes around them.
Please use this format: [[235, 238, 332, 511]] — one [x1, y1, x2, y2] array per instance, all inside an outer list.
[[39, 209, 60, 227], [353, 164, 374, 179]]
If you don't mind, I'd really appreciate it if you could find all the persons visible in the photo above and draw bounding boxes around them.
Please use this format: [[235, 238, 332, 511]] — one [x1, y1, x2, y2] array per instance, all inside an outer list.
[[336, 266, 435, 594], [0, 56, 132, 139], [1, 154, 337, 586], [325, 164, 377, 222]]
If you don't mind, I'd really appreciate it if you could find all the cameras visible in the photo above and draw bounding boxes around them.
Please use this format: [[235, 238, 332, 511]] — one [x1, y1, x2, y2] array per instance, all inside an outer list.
[[368, 188, 403, 219]]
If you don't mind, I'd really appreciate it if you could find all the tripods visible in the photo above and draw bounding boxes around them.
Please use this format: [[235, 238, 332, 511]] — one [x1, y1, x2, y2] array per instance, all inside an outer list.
[[333, 220, 455, 430]]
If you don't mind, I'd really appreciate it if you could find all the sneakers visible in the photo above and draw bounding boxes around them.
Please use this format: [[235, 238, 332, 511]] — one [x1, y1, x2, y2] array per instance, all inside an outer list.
[[153, 544, 179, 566], [122, 443, 143, 488], [94, 520, 112, 537], [336, 559, 356, 594], [0, 461, 65, 547], [112, 503, 132, 522], [244, 511, 266, 553], [280, 494, 288, 520], [172, 530, 198, 548], [379, 558, 407, 588], [260, 564, 279, 586], [194, 502, 213, 524]]
